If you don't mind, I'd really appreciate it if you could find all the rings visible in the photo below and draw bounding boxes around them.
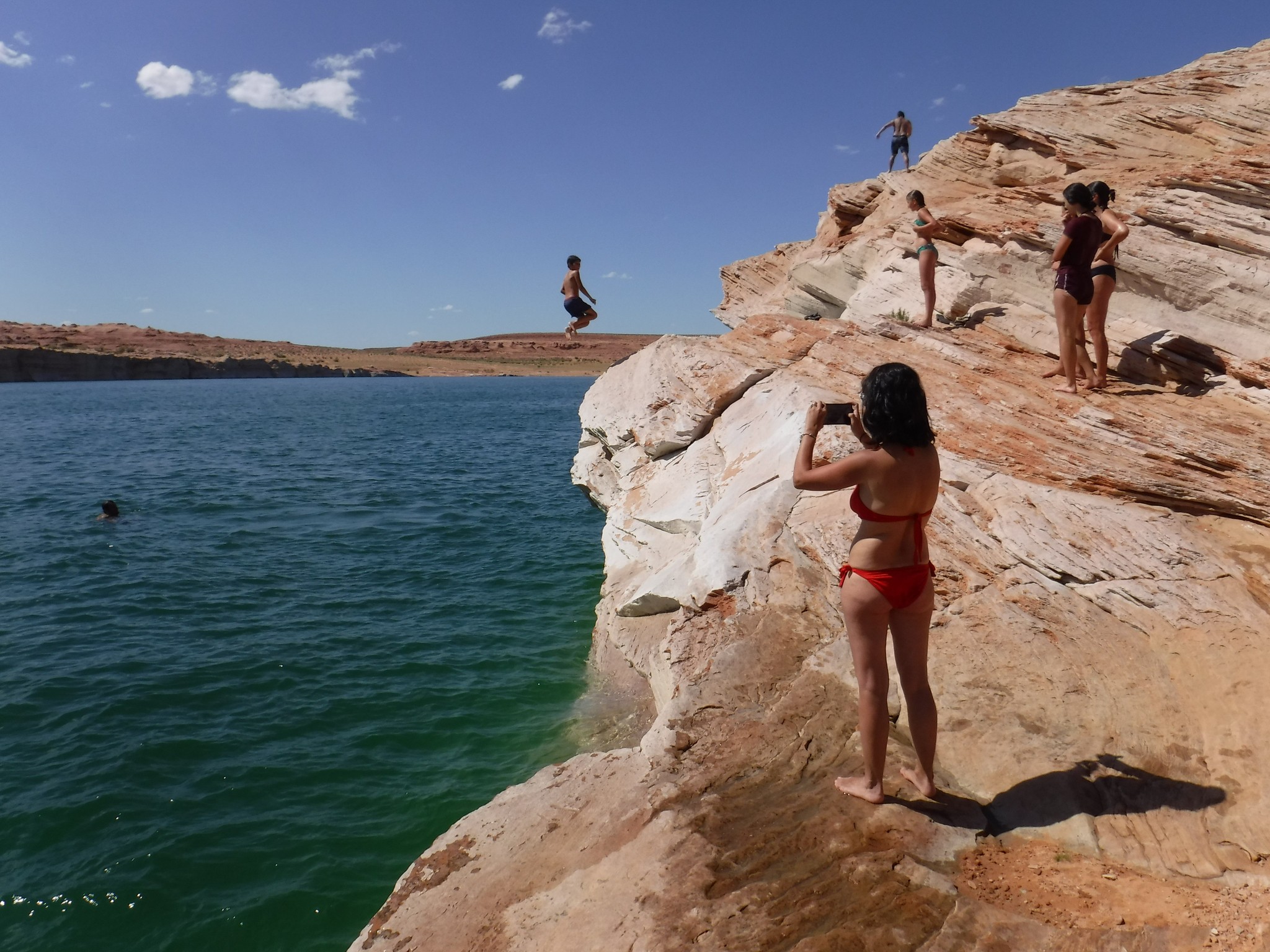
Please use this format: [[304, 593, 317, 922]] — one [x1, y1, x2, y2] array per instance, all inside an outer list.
[[811, 403, 817, 407]]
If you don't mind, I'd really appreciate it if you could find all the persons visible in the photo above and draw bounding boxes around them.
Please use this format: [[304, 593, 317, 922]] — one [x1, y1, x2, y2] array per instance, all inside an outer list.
[[792, 361, 941, 804], [560, 255, 598, 341], [906, 190, 943, 327], [96, 500, 119, 520], [1040, 181, 1129, 393], [876, 111, 916, 173]]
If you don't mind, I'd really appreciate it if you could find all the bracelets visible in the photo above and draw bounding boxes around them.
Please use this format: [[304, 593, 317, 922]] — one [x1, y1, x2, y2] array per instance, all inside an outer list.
[[799, 433, 816, 448]]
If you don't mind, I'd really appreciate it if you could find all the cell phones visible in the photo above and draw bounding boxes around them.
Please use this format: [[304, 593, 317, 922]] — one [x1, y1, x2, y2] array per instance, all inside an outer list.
[[822, 403, 853, 425]]
